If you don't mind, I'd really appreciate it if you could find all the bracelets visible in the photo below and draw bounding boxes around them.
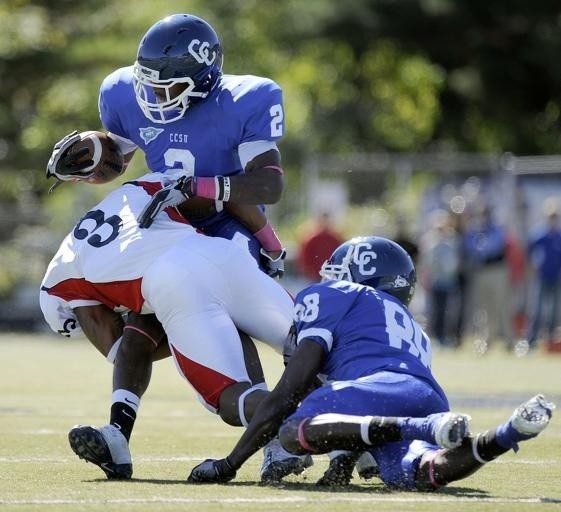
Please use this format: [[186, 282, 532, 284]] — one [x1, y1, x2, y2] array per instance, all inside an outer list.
[[214, 174, 232, 203]]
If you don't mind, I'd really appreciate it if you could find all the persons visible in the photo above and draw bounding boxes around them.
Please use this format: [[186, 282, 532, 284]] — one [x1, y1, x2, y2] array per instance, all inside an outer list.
[[37, 165, 327, 434], [184, 234, 555, 494], [46, 12, 289, 478]]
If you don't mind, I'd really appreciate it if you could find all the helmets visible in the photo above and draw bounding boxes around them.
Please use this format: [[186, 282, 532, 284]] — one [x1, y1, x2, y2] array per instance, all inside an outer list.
[[320, 235, 418, 310], [134, 14, 223, 106]]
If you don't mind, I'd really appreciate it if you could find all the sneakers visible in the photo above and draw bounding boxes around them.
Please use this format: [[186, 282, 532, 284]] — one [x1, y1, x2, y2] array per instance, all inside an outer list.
[[68, 423, 133, 481], [508, 394, 555, 438], [426, 411, 472, 450], [187, 459, 237, 482], [260, 436, 313, 482]]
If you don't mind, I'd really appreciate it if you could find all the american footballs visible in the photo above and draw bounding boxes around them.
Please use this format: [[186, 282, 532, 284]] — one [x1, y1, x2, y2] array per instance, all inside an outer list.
[[66, 130, 124, 184]]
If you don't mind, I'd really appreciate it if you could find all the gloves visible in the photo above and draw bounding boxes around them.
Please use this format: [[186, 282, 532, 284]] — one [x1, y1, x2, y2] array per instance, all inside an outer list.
[[45, 130, 96, 181], [137, 176, 194, 229], [255, 247, 286, 279]]
